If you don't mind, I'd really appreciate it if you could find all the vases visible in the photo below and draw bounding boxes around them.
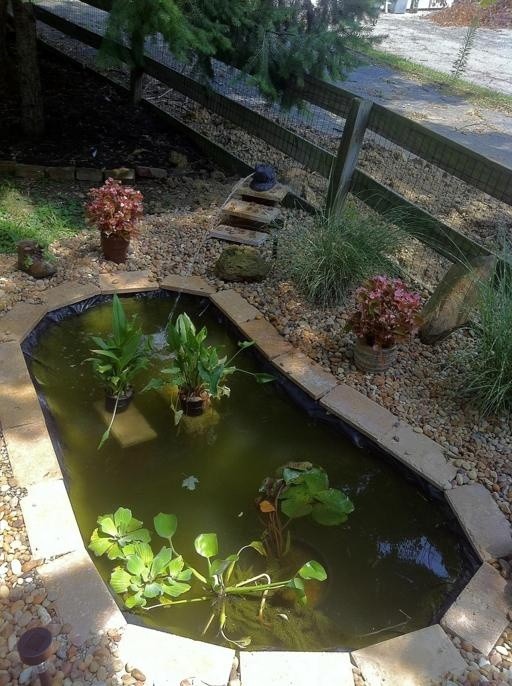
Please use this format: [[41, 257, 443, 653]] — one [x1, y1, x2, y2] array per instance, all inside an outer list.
[[354, 339, 399, 374], [100, 234, 129, 263]]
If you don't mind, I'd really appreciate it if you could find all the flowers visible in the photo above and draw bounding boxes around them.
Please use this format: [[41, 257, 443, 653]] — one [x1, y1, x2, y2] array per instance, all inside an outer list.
[[338, 273, 424, 353], [84, 177, 144, 243]]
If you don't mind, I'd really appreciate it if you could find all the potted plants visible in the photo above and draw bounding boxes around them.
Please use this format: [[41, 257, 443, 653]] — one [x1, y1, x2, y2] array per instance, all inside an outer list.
[[79, 288, 279, 452], [232, 461, 355, 615]]
